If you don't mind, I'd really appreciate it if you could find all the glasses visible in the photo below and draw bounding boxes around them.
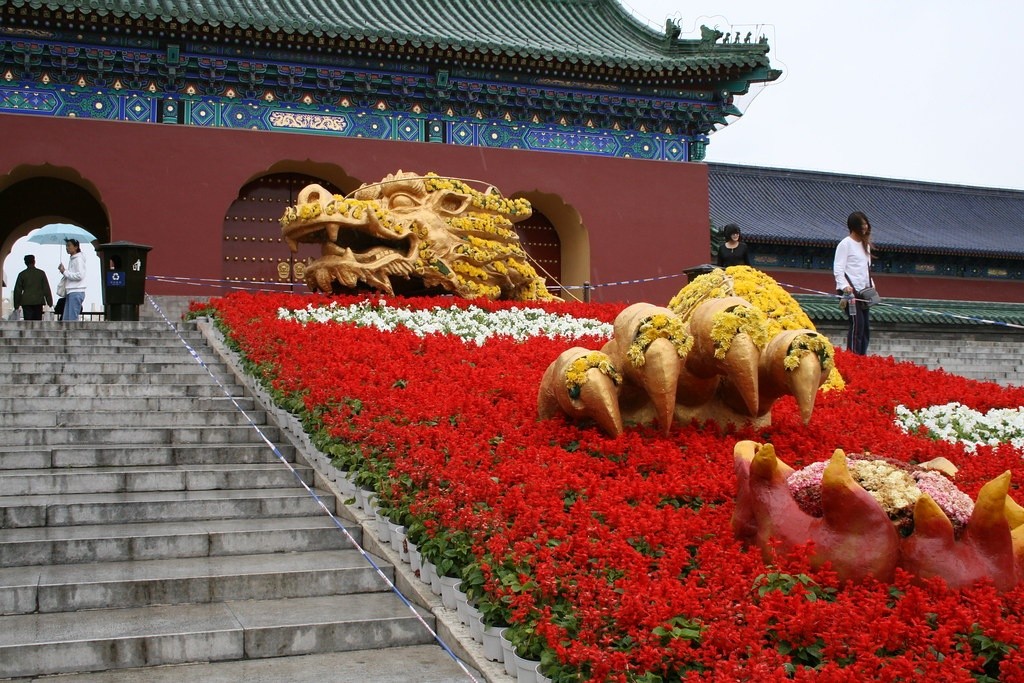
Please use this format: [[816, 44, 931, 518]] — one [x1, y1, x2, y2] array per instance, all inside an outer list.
[[861, 224, 868, 229]]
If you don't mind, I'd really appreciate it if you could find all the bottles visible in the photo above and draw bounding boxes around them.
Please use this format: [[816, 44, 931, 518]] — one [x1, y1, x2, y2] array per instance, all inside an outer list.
[[838, 291, 848, 309], [848, 294, 857, 315]]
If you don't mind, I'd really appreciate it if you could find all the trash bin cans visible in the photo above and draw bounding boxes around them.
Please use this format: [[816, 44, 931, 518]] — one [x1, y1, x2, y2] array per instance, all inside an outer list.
[[682, 263, 726, 285], [94, 239, 155, 322]]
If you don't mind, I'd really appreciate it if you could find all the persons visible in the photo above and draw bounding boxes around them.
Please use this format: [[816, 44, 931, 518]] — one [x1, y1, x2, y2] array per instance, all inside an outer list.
[[716, 224, 749, 268], [54, 297, 83, 321], [58, 239, 88, 320], [833, 212, 875, 356], [13, 255, 53, 320]]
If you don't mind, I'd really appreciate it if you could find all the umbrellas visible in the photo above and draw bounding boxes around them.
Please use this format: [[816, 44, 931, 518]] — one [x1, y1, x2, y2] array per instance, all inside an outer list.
[[26, 223, 97, 264]]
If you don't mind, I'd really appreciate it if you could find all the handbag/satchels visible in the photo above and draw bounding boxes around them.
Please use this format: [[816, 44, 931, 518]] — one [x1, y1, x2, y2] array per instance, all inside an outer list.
[[56, 276, 67, 297], [54, 297, 83, 314], [858, 287, 881, 310], [8, 306, 24, 320]]
